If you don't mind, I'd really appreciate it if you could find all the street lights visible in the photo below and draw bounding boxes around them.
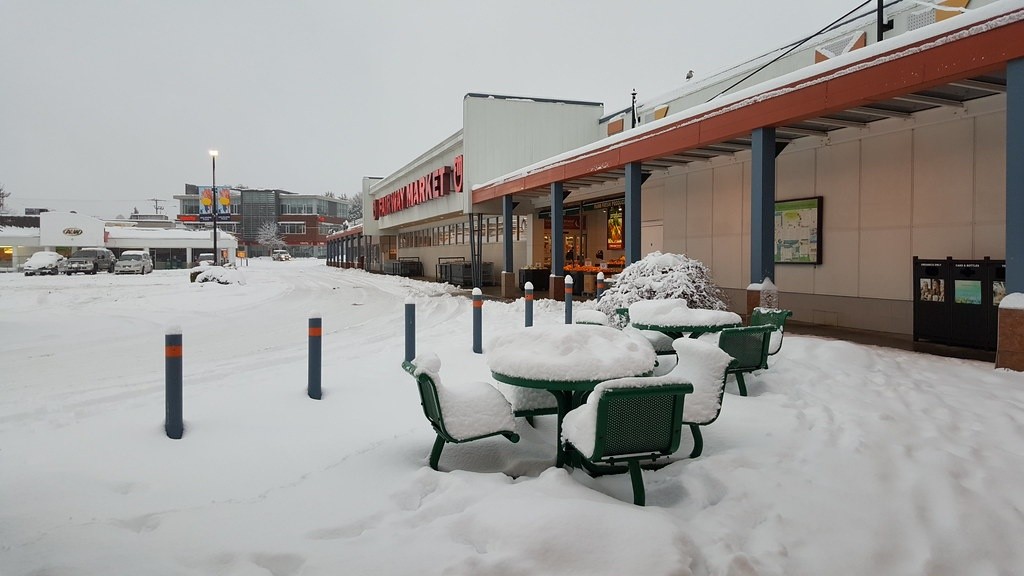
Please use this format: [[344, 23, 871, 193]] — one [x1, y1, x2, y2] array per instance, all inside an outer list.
[[209, 150, 219, 265]]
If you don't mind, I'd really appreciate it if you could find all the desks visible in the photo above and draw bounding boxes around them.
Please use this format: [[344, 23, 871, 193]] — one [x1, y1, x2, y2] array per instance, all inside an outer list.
[[490, 370, 654, 476], [632, 322, 742, 365]]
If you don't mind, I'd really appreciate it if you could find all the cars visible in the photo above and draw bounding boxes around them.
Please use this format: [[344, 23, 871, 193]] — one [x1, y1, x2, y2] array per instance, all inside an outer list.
[[24, 251, 67, 276], [114, 250, 153, 275], [196, 253, 215, 266], [60, 247, 117, 275]]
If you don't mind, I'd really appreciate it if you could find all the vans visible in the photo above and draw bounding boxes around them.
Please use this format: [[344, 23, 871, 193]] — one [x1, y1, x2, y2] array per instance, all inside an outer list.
[[272, 250, 292, 261]]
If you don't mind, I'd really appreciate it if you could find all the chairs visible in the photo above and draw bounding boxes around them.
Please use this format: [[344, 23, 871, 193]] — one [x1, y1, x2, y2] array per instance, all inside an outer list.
[[401, 361, 520, 472], [747, 307, 793, 374], [677, 354, 739, 460], [563, 384, 695, 507], [719, 324, 777, 397]]
